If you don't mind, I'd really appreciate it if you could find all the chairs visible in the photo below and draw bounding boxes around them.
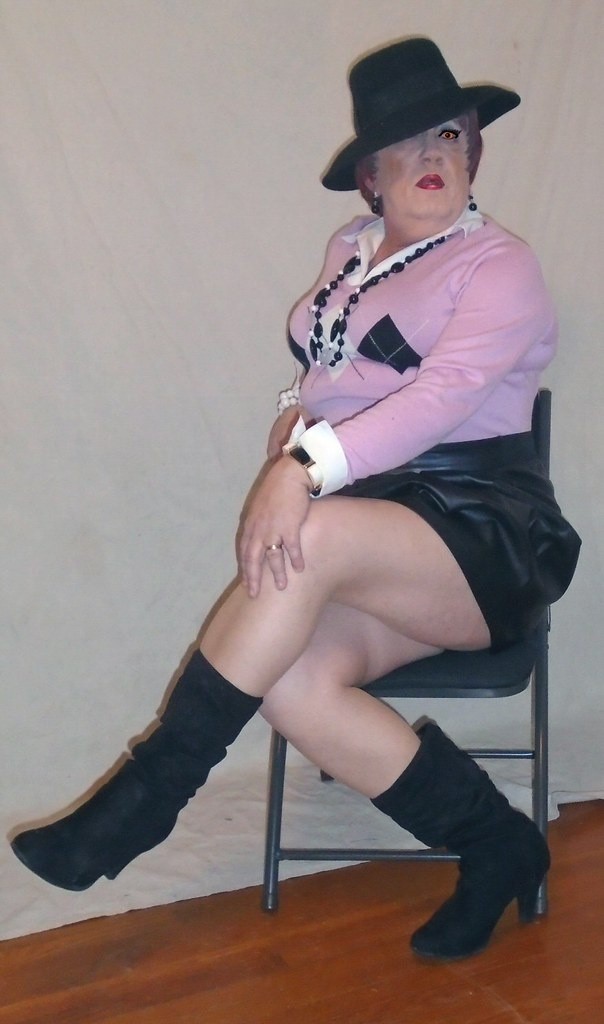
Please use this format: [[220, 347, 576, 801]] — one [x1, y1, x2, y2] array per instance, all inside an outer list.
[[261, 389, 547, 917]]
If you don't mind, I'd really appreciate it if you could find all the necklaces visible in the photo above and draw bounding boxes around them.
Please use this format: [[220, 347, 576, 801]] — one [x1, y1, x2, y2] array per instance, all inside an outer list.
[[307, 233, 461, 367]]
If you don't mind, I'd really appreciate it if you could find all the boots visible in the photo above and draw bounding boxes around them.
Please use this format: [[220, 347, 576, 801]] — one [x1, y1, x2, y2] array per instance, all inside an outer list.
[[10, 647, 264, 891], [371, 723, 551, 958]]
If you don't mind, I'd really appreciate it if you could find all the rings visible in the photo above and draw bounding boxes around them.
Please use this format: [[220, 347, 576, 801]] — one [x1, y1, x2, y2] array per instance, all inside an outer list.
[[266, 545, 284, 551]]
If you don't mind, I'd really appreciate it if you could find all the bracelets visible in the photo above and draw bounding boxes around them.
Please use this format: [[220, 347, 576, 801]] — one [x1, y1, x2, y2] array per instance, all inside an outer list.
[[277, 389, 302, 415], [280, 440, 324, 498]]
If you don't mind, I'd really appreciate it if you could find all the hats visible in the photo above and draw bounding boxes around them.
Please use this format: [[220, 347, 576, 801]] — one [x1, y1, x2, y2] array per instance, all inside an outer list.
[[321, 38, 520, 191]]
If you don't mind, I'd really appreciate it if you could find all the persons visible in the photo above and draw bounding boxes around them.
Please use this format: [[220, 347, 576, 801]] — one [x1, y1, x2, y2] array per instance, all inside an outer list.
[[12, 37, 584, 957]]
